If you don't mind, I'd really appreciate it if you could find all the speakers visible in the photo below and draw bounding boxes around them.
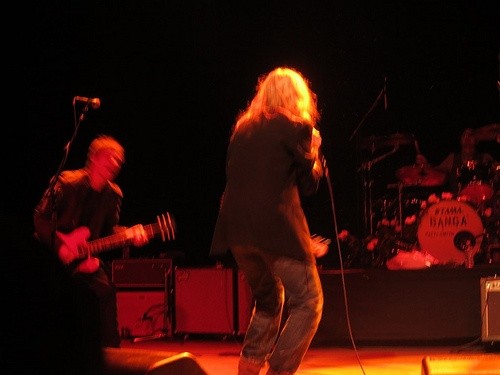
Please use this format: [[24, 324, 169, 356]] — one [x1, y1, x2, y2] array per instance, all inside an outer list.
[[171, 264, 235, 338], [480, 274, 500, 345], [115, 284, 171, 341]]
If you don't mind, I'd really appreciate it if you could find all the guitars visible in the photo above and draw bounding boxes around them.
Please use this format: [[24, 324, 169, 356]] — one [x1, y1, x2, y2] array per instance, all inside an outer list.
[[54, 212, 175, 276]]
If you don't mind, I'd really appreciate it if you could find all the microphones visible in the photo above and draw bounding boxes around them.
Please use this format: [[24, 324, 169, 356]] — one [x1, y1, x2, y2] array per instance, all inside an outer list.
[[75, 95, 102, 110], [383, 77, 388, 110]]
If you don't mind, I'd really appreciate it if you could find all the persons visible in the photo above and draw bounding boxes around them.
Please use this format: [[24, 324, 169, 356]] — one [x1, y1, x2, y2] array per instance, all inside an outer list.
[[418, 126, 500, 186], [209, 67, 330, 375], [33, 136, 150, 287]]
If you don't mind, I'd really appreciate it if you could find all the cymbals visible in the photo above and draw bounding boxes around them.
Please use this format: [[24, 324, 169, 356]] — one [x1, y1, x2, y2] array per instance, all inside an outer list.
[[367, 134, 420, 144], [396, 165, 447, 186]]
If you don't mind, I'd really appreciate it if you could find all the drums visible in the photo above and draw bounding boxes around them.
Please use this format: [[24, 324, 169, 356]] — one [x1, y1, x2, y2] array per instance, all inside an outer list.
[[417, 196, 486, 266], [456, 156, 493, 203], [386, 200, 423, 240]]
